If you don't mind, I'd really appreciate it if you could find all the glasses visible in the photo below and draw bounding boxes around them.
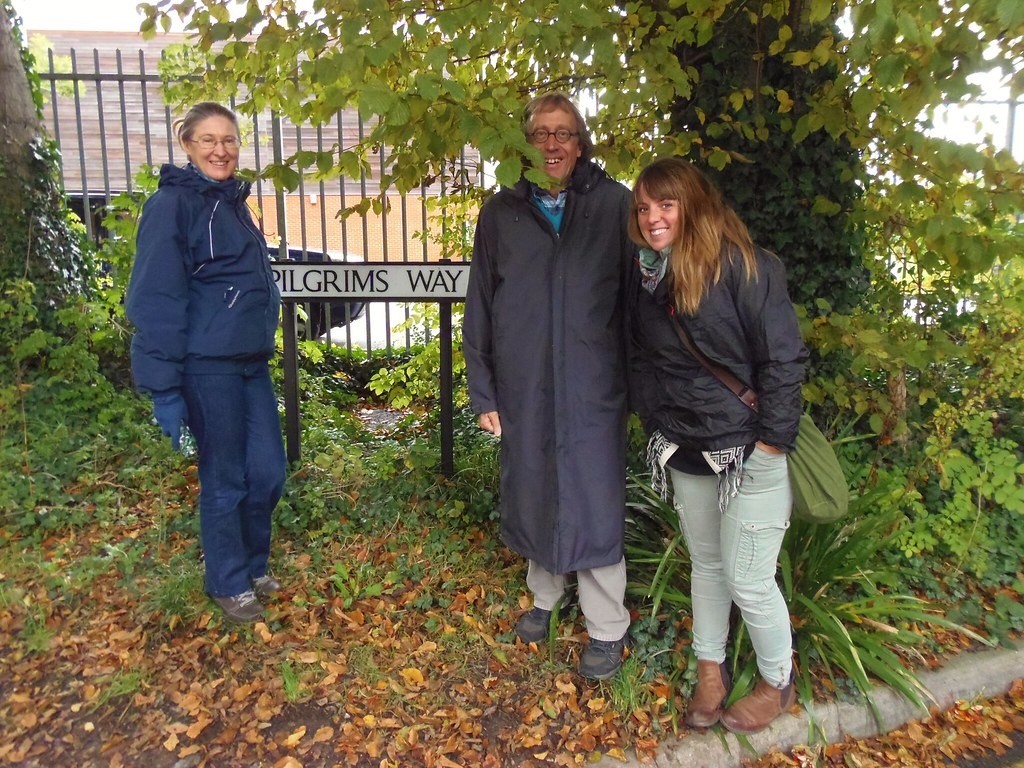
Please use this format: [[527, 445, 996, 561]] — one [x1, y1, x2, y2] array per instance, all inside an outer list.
[[188, 136, 242, 149], [528, 130, 580, 143]]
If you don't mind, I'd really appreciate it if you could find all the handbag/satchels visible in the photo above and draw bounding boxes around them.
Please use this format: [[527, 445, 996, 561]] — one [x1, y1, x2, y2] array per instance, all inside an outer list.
[[781, 412, 849, 525]]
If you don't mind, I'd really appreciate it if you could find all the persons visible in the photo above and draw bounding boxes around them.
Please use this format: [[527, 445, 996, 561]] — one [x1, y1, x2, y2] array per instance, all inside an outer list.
[[125, 103, 287, 623], [461, 94, 636, 680], [625, 159, 805, 734]]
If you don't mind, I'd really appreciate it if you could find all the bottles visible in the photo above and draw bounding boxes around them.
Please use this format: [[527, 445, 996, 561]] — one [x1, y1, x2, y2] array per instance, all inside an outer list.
[[177, 420, 199, 459]]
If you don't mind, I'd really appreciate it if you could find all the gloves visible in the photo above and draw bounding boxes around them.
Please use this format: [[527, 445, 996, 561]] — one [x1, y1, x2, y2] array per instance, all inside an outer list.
[[154, 398, 189, 451]]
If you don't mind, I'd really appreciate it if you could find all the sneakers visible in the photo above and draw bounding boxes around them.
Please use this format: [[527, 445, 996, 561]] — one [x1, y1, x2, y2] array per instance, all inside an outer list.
[[577, 631, 629, 680], [210, 586, 264, 621], [514, 604, 569, 644], [253, 575, 281, 594]]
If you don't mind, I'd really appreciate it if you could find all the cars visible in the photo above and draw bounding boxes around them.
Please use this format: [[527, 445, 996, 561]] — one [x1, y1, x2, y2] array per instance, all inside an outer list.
[[61, 186, 375, 343]]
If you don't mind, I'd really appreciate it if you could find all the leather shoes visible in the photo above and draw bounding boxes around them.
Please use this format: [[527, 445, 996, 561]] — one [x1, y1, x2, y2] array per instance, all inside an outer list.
[[685, 658, 731, 725], [719, 670, 795, 736]]
